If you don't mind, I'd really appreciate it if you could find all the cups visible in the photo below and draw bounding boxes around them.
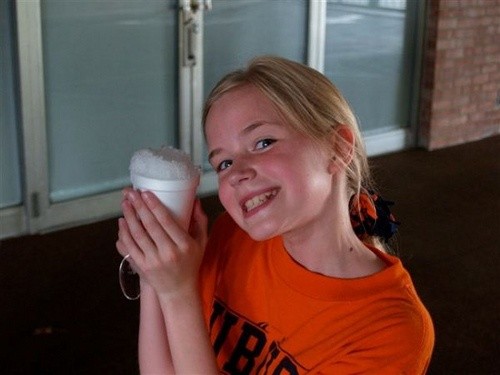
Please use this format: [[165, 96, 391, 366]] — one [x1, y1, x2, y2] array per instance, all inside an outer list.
[[128, 165, 200, 234]]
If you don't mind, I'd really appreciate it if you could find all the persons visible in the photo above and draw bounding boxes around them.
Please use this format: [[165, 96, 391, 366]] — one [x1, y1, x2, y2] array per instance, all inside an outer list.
[[115, 55, 435, 374]]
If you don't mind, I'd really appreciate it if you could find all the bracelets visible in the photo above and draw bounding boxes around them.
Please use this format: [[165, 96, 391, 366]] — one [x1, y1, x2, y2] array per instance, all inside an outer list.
[[118, 253, 142, 301]]
